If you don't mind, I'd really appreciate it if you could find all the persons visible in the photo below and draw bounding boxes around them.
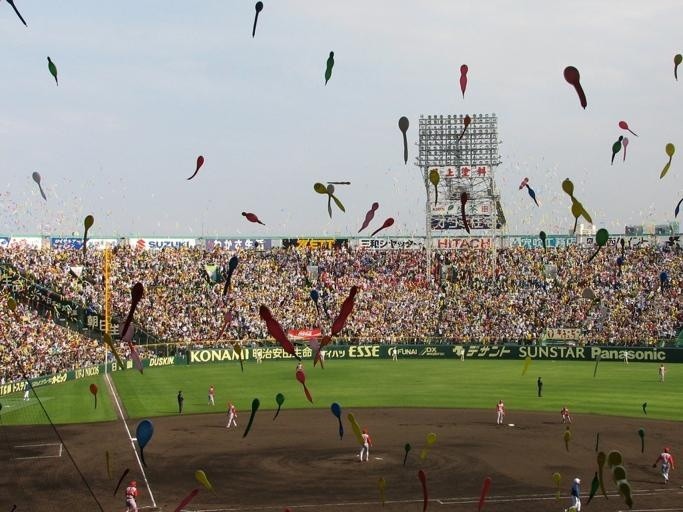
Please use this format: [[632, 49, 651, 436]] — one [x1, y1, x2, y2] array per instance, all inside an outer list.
[[1, 236, 682, 391], [651, 447, 675, 484]]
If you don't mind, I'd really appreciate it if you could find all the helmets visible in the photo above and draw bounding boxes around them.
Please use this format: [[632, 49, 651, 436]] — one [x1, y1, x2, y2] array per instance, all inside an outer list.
[[574, 478, 581, 484], [128, 480, 136, 487], [664, 448, 670, 452]]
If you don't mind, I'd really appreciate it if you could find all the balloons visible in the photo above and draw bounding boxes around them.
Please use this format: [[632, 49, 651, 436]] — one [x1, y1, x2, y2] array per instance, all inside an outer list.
[[45, 55, 58, 86], [102, 392, 648, 512], [250, 1, 265, 37], [187, 153, 204, 182], [306, 50, 682, 262], [23, 384, 31, 401], [241, 212, 265, 227], [80, 214, 93, 254], [87, 383, 98, 409], [31, 172, 47, 203], [7, 0, 27, 31]]
